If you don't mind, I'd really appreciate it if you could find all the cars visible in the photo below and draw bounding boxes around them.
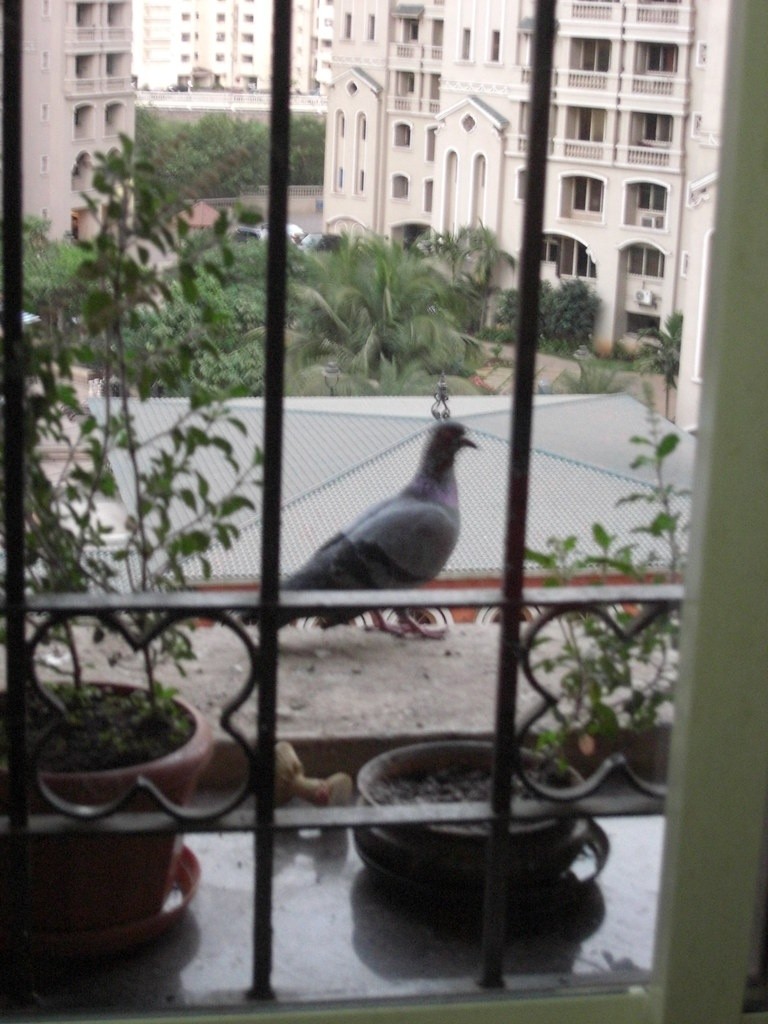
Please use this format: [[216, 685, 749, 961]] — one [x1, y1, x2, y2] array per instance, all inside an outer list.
[[236, 220, 306, 246], [295, 231, 343, 255]]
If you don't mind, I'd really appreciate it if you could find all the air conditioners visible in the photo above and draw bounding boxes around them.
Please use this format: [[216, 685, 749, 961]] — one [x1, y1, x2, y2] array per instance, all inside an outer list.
[[635, 289, 652, 306]]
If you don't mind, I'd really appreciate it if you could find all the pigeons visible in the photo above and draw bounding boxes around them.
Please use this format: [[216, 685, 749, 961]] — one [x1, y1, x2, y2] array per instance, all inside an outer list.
[[238, 419, 483, 638]]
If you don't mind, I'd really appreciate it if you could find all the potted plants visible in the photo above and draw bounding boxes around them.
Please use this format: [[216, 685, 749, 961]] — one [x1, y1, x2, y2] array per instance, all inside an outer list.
[[0, 114, 269, 959], [351, 381, 704, 906]]
